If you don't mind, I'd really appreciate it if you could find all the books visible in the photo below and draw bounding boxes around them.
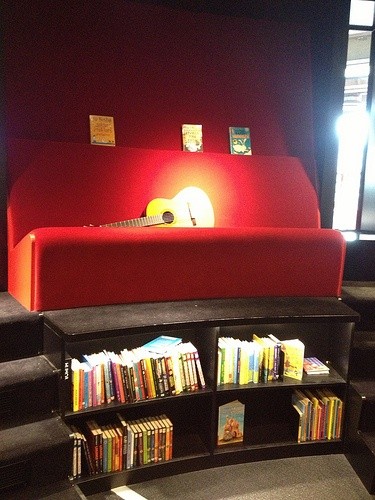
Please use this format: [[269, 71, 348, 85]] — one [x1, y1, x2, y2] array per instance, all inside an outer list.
[[181, 124, 204, 153], [66, 331, 345, 482], [228, 126, 253, 157], [89, 114, 116, 148]]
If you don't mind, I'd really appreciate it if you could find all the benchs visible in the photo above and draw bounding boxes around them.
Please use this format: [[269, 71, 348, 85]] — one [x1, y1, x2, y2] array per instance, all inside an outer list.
[[3, 134, 346, 312]]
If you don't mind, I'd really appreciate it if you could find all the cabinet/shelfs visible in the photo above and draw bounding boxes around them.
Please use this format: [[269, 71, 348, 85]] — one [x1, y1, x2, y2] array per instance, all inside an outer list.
[[42, 301, 363, 490]]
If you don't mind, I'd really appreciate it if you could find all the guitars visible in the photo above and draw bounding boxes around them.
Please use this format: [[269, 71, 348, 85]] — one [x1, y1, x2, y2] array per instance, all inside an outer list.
[[79, 185, 216, 229]]
